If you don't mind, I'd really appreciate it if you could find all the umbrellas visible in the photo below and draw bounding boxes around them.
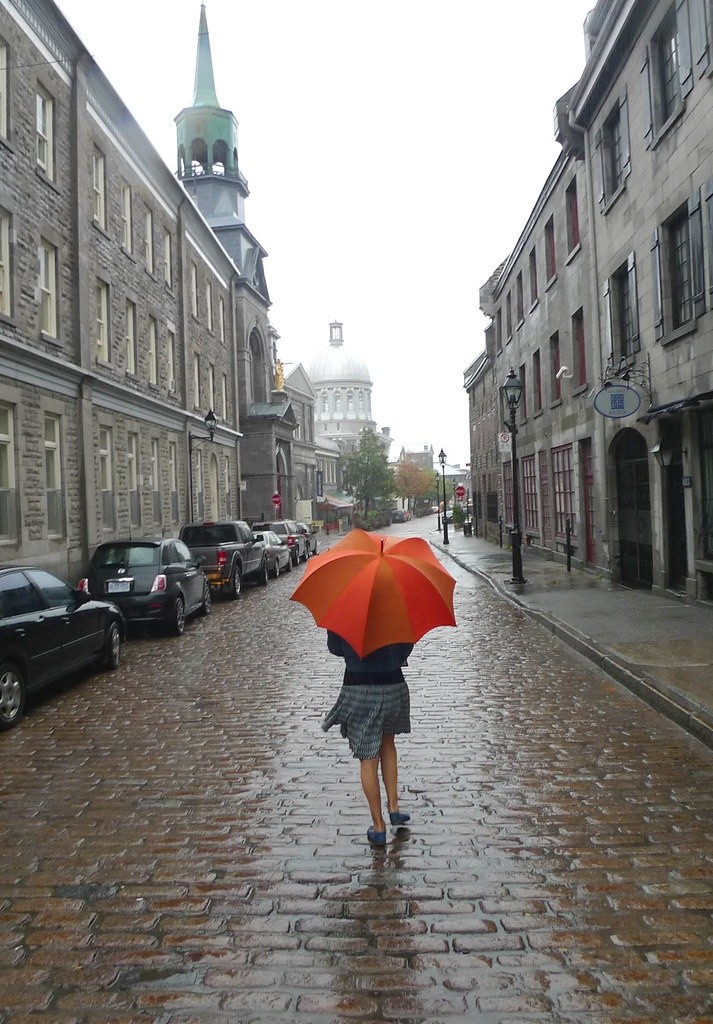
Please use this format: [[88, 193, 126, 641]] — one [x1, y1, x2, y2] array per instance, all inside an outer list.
[[288, 528, 457, 661]]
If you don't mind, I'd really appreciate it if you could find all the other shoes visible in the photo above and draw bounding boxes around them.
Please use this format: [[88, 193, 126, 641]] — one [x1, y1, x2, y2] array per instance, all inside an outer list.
[[390, 812, 410, 826], [367, 826, 386, 845]]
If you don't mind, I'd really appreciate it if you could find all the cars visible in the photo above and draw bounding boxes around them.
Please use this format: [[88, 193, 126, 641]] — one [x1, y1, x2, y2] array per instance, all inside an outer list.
[[296, 522, 318, 557], [74, 537, 212, 638], [0, 563, 129, 731], [250, 529, 293, 578]]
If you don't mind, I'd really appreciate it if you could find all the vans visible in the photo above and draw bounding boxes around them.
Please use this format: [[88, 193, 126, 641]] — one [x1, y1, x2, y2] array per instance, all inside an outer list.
[[392, 509, 411, 523]]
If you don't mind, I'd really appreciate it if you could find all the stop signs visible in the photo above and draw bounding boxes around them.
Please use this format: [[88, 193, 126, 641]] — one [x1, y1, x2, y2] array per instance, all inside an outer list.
[[271, 494, 282, 505], [455, 486, 467, 497]]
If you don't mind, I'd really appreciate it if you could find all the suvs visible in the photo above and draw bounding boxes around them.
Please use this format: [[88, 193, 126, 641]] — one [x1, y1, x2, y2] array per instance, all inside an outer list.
[[251, 519, 311, 566]]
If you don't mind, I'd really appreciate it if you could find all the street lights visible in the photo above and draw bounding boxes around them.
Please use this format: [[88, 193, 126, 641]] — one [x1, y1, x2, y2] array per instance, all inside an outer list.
[[498, 367, 527, 584], [438, 448, 449, 545], [323, 497, 330, 536], [435, 472, 442, 532], [187, 409, 219, 523], [452, 478, 457, 506]]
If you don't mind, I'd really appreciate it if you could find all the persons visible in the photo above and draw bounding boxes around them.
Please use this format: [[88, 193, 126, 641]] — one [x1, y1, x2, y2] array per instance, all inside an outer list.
[[322, 629, 414, 846]]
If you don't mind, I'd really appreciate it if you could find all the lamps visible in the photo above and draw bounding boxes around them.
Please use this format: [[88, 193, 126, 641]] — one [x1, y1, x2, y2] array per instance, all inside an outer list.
[[191, 409, 219, 453], [650, 434, 688, 469]]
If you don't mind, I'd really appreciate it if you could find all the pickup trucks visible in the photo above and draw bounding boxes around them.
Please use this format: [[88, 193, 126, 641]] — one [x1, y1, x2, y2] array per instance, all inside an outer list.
[[176, 519, 269, 600]]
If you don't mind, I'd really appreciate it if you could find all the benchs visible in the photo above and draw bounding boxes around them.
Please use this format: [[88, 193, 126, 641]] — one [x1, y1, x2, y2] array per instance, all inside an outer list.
[[312, 520, 325, 533]]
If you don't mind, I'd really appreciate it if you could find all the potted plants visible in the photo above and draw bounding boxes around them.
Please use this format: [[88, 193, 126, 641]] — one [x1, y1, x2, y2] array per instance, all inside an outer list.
[[452, 503, 465, 528]]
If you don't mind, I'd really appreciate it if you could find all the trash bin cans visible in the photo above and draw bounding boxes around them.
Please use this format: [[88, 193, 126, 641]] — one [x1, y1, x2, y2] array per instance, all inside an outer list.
[[463, 523, 472, 537]]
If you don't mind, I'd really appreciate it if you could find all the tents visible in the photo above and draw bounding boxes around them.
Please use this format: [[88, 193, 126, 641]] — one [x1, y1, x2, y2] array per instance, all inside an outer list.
[[318, 493, 361, 534]]
